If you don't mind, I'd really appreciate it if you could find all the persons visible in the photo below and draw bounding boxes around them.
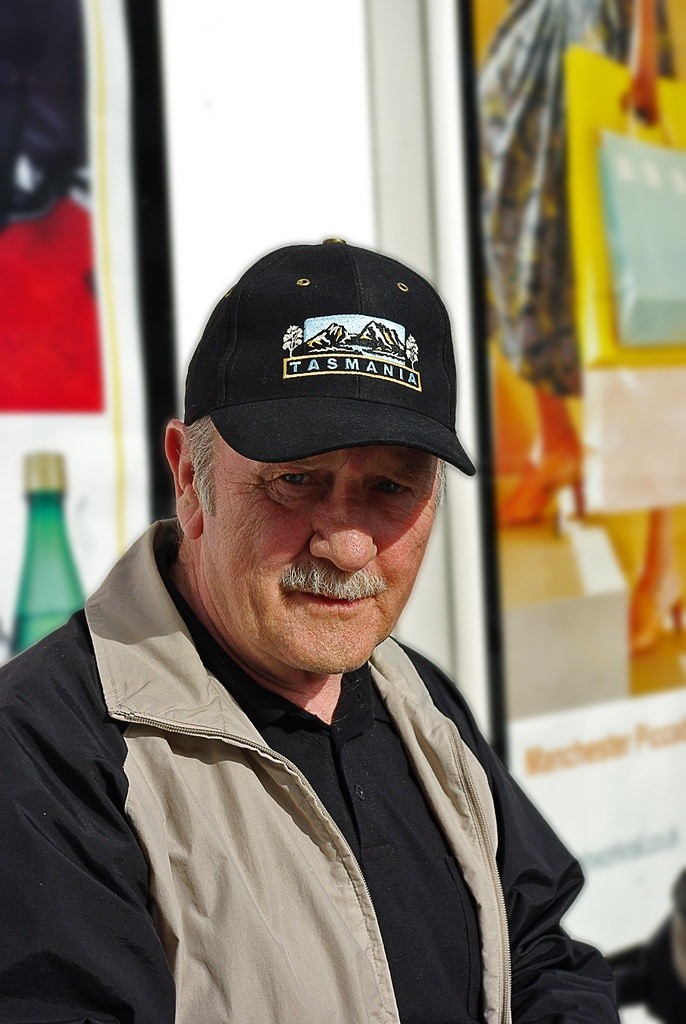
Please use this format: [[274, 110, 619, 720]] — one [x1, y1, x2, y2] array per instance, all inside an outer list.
[[0, 238, 622, 1024]]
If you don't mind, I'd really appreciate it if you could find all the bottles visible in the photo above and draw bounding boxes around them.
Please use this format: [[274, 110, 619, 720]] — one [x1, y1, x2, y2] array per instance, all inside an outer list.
[[12, 450, 88, 658]]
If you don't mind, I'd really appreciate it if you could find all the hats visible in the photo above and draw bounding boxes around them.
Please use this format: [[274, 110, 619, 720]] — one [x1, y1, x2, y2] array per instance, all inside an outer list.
[[183, 240, 477, 476]]
